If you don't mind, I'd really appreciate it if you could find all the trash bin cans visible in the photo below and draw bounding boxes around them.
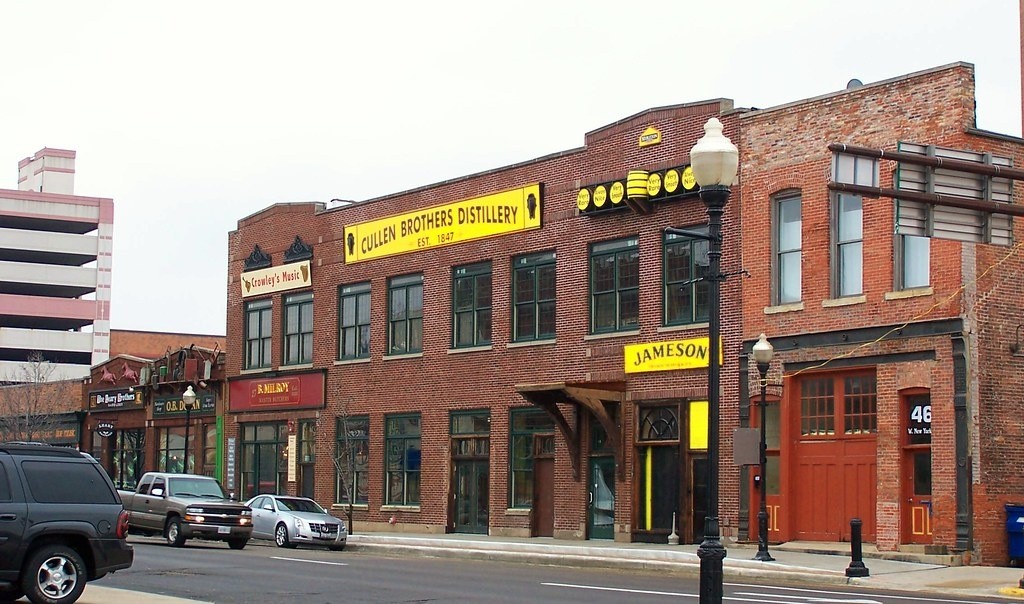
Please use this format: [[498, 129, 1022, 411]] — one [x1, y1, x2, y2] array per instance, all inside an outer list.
[[1005, 502, 1024, 567]]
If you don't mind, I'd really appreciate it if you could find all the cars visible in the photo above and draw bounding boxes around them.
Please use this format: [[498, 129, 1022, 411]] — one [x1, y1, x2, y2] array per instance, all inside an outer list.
[[245, 495, 347, 550], [0, 440, 134, 603]]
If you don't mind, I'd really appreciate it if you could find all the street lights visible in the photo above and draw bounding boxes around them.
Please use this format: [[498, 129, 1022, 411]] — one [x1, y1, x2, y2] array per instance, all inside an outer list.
[[665, 118, 746, 603], [752, 333, 785, 562], [183, 385, 195, 473]]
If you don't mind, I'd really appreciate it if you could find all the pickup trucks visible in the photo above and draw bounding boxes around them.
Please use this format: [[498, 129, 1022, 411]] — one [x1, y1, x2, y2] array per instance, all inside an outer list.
[[117, 471, 253, 549]]
